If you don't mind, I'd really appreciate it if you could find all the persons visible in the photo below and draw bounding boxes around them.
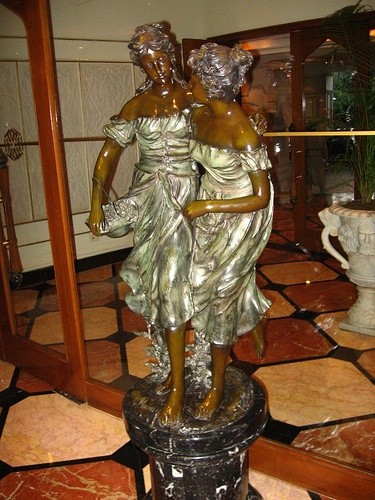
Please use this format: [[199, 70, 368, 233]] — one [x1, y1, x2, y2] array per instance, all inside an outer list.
[[85, 20, 270, 432], [181, 40, 279, 424]]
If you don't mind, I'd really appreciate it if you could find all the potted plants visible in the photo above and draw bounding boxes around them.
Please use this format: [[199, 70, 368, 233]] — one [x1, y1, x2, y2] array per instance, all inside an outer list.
[[314, 0, 375, 338]]
